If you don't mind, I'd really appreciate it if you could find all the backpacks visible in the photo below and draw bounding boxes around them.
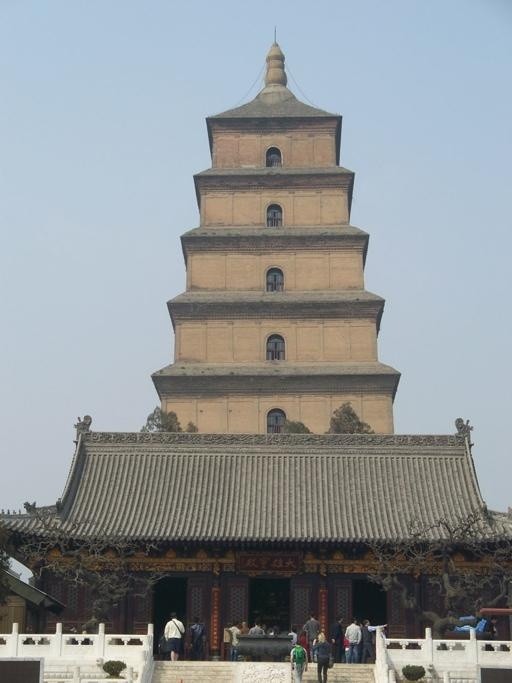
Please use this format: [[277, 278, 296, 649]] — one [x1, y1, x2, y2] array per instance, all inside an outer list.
[[294, 645, 305, 665]]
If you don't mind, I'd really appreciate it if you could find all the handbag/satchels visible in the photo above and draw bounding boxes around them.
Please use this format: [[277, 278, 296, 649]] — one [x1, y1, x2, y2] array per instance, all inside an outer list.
[[181, 634, 184, 638], [328, 653, 334, 668]]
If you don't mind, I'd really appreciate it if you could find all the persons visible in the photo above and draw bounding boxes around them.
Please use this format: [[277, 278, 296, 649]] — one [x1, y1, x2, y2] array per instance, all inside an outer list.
[[484, 615, 499, 640], [329, 614, 388, 664], [310, 632, 332, 682], [290, 640, 308, 683], [228, 619, 280, 662], [288, 625, 297, 655], [164, 611, 185, 661], [299, 612, 321, 663], [475, 617, 487, 633], [189, 615, 207, 661]]
